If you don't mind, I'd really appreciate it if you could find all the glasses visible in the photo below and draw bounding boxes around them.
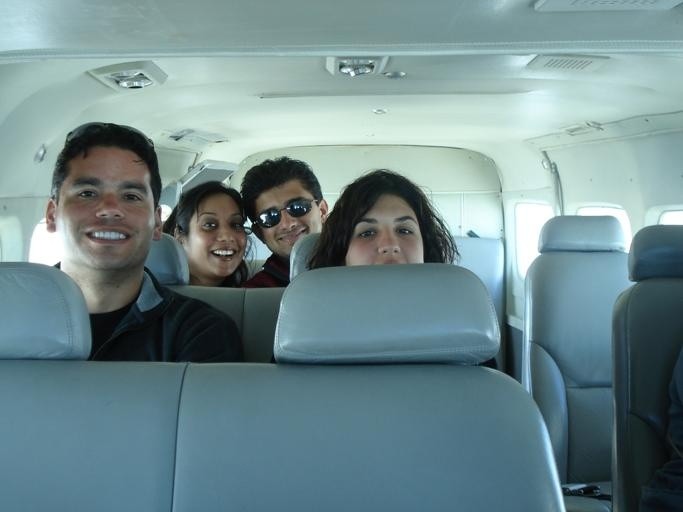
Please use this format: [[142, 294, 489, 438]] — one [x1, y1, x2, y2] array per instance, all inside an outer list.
[[253, 198, 319, 230], [65, 120, 156, 147]]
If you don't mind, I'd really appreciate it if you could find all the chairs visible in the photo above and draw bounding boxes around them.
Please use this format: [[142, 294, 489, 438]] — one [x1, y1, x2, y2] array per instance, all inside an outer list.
[[519, 213, 629, 512], [0, 212, 568, 511], [608, 221, 682, 512]]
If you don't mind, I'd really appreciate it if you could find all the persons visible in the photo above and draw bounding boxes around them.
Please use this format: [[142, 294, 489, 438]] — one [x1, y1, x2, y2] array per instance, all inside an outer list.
[[160, 179, 247, 287], [308, 169, 457, 266], [241, 157, 328, 289], [41, 122, 245, 364]]
